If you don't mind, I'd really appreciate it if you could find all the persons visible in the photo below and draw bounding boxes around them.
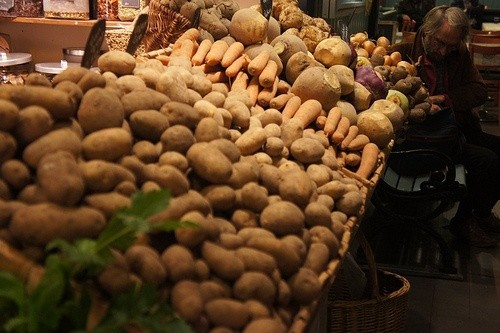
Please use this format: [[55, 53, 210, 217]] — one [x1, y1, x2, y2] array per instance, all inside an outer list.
[[390, 0, 500, 248]]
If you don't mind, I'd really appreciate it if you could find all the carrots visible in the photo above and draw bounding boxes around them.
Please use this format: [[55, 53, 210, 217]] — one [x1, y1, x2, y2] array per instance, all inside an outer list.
[[153, 28, 381, 181]]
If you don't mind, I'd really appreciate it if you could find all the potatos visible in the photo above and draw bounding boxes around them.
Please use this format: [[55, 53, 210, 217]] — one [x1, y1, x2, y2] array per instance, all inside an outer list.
[[158, 0, 435, 120], [0, 50, 363, 333]]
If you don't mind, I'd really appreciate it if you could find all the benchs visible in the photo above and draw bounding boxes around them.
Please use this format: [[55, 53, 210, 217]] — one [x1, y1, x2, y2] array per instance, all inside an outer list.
[[366, 148, 464, 281]]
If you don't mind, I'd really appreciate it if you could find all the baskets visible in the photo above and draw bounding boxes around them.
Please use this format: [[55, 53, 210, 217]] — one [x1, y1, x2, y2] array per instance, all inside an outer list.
[[145, 0, 192, 53], [327, 230, 410, 333]]
